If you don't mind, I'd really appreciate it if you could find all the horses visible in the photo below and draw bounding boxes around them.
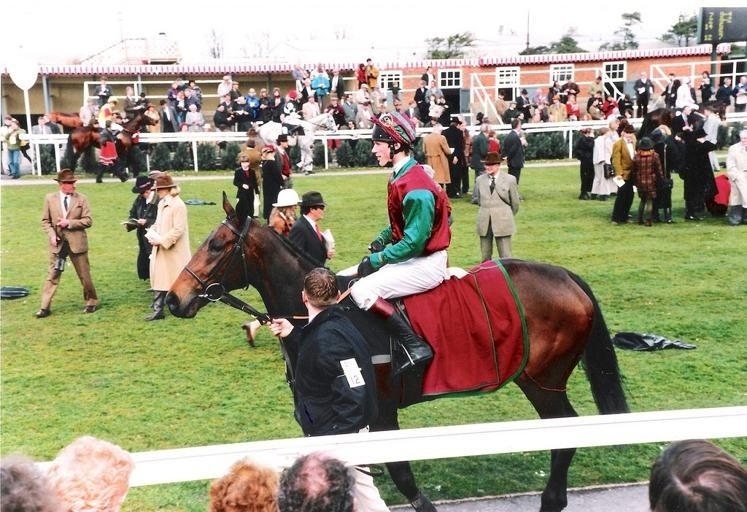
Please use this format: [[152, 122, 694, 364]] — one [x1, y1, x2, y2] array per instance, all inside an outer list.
[[62, 112, 159, 184], [41, 108, 83, 135], [247, 112, 338, 176], [165, 190, 630, 512], [637, 99, 727, 139]]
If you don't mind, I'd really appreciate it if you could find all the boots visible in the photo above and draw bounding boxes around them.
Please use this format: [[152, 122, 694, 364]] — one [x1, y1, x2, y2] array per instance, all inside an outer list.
[[365, 295, 432, 378]]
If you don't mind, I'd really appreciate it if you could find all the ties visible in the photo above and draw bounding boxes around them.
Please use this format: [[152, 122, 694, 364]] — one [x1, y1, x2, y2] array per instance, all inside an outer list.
[[315, 223, 323, 244], [64, 197, 69, 212], [489, 176, 495, 194]]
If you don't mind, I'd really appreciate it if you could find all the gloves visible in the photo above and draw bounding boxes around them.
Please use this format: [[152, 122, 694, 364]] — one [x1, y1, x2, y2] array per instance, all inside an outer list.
[[356, 255, 377, 277], [367, 240, 385, 253]]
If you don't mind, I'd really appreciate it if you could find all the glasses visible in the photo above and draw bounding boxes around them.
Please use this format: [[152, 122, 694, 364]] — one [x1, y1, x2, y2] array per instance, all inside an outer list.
[[310, 205, 325, 211]]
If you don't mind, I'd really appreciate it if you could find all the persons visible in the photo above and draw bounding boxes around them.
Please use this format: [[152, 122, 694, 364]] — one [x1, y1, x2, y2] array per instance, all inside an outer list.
[[145, 174, 193, 321], [648, 438, 747, 512], [336, 109, 455, 310], [242, 188, 302, 347], [122, 175, 162, 280], [289, 189, 336, 265], [267, 267, 391, 512], [470, 152, 520, 262], [32, 168, 99, 319]]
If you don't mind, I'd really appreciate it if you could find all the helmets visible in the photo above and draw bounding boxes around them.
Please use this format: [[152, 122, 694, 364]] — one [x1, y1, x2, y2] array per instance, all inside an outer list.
[[363, 109, 418, 151]]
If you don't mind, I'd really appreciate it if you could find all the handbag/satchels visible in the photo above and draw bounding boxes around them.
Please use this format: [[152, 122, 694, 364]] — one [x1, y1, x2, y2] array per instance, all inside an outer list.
[[603, 163, 615, 179], [655, 174, 675, 191]]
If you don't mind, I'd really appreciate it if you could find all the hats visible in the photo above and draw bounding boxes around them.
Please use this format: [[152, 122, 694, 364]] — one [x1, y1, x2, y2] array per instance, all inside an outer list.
[[282, 88, 340, 102], [638, 138, 655, 150], [276, 134, 288, 143], [246, 128, 258, 147], [650, 129, 667, 143], [296, 191, 327, 208], [150, 173, 177, 192], [52, 168, 78, 183], [624, 123, 635, 133], [222, 75, 240, 86], [478, 151, 505, 164], [499, 86, 561, 106], [448, 116, 462, 126], [131, 175, 155, 194], [270, 188, 302, 208], [140, 92, 168, 109]]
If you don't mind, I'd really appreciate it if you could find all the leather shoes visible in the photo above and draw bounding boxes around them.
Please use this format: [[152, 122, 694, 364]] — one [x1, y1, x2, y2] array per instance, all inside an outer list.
[[144, 312, 167, 321], [83, 305, 97, 314], [240, 324, 257, 349], [35, 309, 51, 319]]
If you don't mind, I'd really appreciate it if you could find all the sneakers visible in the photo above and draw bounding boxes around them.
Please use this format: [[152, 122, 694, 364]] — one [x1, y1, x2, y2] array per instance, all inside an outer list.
[[578, 192, 608, 202], [8, 172, 21, 180], [94, 174, 129, 183], [607, 213, 710, 228]]
